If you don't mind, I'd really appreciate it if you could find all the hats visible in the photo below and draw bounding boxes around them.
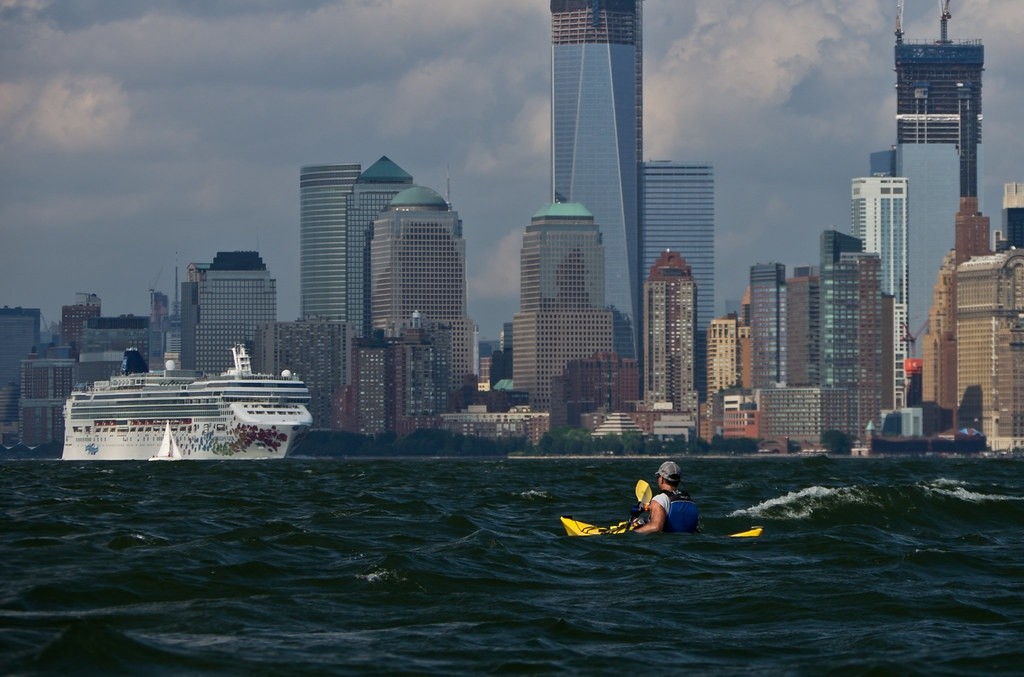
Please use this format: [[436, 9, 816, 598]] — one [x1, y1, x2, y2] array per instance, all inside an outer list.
[[655, 461, 682, 482]]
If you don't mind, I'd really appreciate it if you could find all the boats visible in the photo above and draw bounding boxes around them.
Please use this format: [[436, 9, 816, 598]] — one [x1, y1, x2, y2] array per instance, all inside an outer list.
[[560, 515, 765, 537], [61, 343, 314, 460]]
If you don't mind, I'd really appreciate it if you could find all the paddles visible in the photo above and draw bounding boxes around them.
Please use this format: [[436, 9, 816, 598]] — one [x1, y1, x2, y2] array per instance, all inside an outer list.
[[621, 479, 653, 533]]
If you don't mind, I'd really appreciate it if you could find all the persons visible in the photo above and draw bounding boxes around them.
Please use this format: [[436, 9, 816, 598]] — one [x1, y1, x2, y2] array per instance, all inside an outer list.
[[632, 460, 698, 535]]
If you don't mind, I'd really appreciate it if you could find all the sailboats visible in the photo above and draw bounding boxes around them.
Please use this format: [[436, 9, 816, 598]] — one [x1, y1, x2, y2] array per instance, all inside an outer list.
[[150, 419, 182, 460]]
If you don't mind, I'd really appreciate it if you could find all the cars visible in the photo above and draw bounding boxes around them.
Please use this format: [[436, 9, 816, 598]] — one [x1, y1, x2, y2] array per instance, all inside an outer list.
[[757, 440, 784, 454]]
